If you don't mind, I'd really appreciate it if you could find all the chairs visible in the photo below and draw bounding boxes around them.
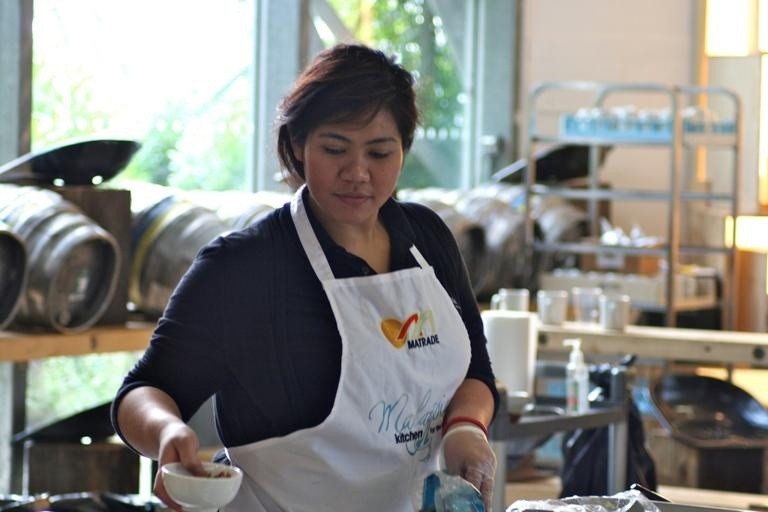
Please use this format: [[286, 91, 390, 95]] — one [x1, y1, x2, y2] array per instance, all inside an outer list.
[[643, 372, 768, 491]]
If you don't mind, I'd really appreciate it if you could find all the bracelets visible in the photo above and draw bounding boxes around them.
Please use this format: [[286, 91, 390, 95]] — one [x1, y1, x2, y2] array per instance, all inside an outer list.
[[440, 415, 489, 434]]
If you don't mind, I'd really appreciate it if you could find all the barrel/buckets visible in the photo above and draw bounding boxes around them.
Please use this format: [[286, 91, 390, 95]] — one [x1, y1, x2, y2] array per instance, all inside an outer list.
[[0, 180, 606, 337]]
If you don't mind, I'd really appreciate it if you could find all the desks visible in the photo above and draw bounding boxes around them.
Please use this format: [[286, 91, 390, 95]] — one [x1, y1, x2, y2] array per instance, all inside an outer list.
[[518, 316, 767, 405]]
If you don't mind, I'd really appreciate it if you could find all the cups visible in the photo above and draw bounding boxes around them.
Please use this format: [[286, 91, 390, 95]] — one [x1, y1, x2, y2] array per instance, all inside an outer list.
[[489, 288, 531, 313], [536, 287, 569, 326], [570, 286, 631, 332]]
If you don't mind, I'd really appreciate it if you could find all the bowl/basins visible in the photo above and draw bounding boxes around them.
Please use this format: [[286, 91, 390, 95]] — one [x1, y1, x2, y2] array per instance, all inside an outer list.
[[159, 462, 243, 512]]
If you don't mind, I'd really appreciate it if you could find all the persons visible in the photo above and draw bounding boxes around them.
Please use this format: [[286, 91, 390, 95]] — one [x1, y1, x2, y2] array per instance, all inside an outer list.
[[106, 39, 502, 512]]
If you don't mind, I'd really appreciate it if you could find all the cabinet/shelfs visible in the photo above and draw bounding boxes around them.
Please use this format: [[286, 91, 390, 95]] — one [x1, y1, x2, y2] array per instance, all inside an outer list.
[[522, 77, 743, 331]]
